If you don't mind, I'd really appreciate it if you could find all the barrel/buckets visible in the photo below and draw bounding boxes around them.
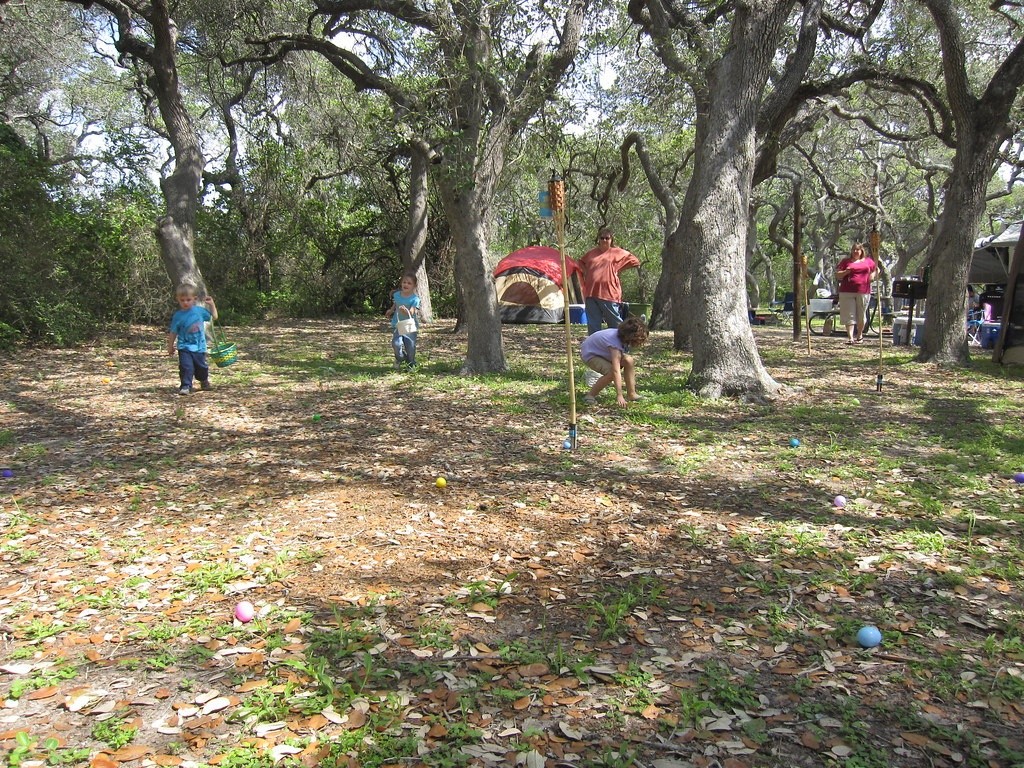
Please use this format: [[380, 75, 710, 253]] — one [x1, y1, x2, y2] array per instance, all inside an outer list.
[[981, 323, 1001, 349], [625, 303, 652, 320], [915, 318, 925, 344], [569, 302, 588, 325], [893, 317, 908, 345]]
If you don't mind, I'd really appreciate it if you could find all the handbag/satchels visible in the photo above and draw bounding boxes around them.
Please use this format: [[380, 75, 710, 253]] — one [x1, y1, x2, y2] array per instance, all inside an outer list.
[[619, 302, 630, 320]]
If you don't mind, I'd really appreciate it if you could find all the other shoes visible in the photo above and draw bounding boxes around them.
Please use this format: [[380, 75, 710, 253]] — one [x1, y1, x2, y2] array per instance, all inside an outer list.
[[393, 359, 401, 370], [201, 379, 211, 391], [178, 384, 189, 395]]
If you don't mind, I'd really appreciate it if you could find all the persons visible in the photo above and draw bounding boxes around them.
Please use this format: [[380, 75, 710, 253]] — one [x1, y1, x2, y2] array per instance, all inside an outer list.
[[836, 244, 880, 344], [168, 284, 218, 395], [576, 229, 640, 336], [386, 273, 420, 371], [581, 316, 649, 408], [968, 284, 994, 343]]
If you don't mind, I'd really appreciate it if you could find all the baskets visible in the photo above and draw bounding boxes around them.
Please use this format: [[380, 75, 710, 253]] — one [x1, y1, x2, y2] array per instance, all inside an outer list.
[[395, 307, 417, 335], [208, 315, 238, 368], [586, 370, 604, 388]]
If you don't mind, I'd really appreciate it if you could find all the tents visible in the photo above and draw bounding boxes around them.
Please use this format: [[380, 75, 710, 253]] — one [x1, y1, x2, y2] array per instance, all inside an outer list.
[[494, 246, 583, 324]]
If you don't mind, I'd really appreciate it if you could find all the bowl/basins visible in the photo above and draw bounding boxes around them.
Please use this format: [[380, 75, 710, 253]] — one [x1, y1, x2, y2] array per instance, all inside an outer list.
[[809, 299, 834, 312]]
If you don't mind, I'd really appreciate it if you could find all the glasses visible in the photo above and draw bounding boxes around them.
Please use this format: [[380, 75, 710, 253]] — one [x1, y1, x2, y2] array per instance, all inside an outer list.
[[600, 236, 611, 240]]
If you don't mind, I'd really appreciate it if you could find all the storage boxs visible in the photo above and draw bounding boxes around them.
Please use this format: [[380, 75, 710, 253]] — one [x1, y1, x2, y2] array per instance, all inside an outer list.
[[809, 299, 834, 311], [893, 317, 925, 346], [982, 323, 1001, 348], [569, 304, 587, 324]]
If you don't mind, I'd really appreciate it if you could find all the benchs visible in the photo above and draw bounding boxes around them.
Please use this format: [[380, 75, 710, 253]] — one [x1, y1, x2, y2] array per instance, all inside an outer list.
[[809, 295, 877, 337]]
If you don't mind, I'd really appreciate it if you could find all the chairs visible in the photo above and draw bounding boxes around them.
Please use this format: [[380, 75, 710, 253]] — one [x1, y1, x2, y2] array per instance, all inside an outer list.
[[968, 309, 985, 346], [768, 292, 805, 326]]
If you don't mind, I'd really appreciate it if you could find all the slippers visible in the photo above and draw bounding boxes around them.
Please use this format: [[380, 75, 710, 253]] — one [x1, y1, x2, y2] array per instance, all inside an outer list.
[[845, 339, 854, 345], [625, 394, 647, 401], [582, 398, 598, 405], [855, 336, 864, 344]]
